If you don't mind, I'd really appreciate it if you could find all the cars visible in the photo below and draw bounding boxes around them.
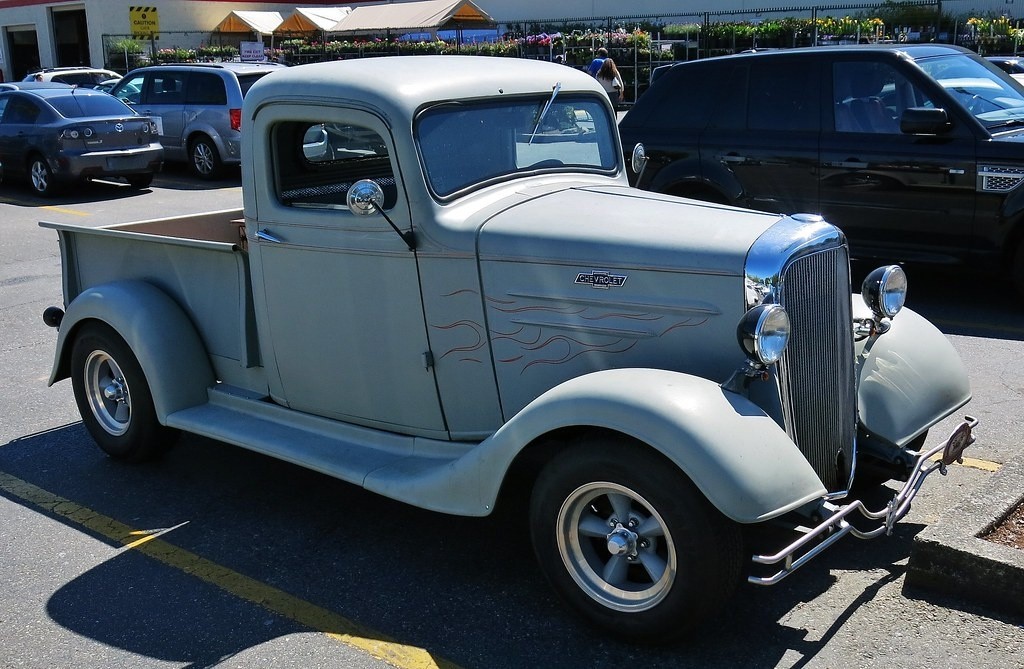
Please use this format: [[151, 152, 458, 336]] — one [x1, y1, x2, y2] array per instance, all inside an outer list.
[[0, 65, 180, 195]]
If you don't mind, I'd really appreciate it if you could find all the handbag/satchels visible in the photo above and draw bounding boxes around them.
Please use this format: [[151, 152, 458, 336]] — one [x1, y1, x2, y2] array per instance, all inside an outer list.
[[612, 71, 621, 90]]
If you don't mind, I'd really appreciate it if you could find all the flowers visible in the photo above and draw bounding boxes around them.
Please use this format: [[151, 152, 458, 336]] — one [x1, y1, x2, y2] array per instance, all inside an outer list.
[[701, 17, 883, 38], [157, 26, 650, 56], [965, 14, 1024, 48]]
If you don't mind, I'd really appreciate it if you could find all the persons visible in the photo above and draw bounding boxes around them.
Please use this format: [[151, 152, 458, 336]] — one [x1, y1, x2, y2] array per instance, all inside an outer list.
[[588, 48, 608, 77], [596, 58, 624, 120]]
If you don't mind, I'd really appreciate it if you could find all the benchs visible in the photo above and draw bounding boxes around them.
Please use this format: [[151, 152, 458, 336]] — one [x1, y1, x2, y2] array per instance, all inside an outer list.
[[280, 177, 396, 201]]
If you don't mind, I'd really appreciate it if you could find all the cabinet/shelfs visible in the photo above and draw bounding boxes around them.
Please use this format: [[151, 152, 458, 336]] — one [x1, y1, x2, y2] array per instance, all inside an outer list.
[[610, 32, 688, 105], [560, 34, 594, 67], [520, 39, 553, 63], [106, 38, 140, 72]]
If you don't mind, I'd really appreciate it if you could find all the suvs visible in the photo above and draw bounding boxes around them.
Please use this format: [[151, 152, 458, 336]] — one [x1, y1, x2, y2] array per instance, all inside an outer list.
[[104, 61, 330, 184], [613, 42, 1023, 313]]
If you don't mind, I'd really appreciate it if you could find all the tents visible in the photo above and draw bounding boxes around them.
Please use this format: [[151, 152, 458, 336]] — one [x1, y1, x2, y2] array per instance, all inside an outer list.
[[221, 0, 497, 41]]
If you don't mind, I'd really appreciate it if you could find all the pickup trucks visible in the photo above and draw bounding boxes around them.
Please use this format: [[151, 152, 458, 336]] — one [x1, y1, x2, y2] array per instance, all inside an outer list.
[[33, 48, 981, 651]]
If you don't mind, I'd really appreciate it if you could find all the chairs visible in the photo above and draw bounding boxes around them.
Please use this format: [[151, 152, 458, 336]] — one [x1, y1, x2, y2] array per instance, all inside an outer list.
[[833, 61, 901, 133], [160, 77, 179, 103]]
